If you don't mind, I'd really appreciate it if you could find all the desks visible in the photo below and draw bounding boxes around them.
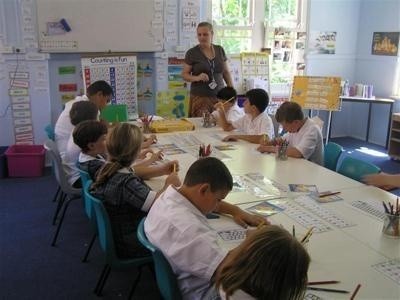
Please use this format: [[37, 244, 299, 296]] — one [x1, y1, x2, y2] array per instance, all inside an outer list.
[[108, 117, 400, 300], [308, 95, 396, 150]]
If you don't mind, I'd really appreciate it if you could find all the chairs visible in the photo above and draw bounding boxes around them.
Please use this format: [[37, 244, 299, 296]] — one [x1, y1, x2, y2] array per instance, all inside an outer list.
[[44, 123, 61, 202], [75, 161, 108, 294], [84, 179, 153, 299], [136, 215, 186, 300], [44, 139, 84, 247], [324, 141, 342, 173], [335, 156, 382, 185]]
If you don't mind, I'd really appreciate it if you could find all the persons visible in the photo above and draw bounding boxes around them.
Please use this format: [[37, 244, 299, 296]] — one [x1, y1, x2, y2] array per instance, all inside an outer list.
[[144, 158, 270, 300], [202, 225, 309, 299], [184, 22, 234, 117], [87, 121, 182, 260], [359, 173, 400, 190], [55, 81, 324, 189]]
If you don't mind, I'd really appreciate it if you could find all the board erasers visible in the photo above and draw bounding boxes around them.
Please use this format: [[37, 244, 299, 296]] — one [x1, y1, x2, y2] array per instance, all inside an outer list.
[[60, 18, 72, 32]]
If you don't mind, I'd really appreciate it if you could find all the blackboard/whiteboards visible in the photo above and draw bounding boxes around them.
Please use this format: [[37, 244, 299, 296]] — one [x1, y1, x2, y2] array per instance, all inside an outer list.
[[36, 0, 164, 53]]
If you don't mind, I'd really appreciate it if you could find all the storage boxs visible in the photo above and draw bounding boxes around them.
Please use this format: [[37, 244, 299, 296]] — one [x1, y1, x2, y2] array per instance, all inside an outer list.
[[4, 144, 48, 177], [0, 146, 9, 179]]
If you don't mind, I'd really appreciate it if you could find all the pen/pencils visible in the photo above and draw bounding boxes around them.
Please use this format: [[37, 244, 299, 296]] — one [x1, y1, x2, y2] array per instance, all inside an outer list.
[[274, 137, 289, 160], [222, 96, 235, 105], [293, 224, 317, 247], [257, 220, 266, 229], [350, 284, 361, 300], [306, 281, 341, 285], [319, 192, 341, 197], [382, 197, 400, 236], [199, 143, 213, 157], [264, 134, 266, 145], [115, 112, 118, 121], [306, 286, 350, 293], [174, 163, 176, 173], [140, 112, 153, 134]]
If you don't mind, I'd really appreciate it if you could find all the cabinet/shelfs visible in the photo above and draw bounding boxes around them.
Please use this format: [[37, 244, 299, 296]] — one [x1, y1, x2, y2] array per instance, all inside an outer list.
[[388, 112, 400, 162]]
